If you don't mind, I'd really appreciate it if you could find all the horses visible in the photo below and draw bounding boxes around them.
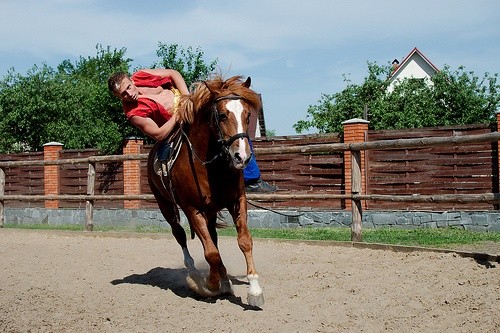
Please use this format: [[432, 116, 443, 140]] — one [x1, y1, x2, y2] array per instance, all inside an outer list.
[[146, 63, 266, 308]]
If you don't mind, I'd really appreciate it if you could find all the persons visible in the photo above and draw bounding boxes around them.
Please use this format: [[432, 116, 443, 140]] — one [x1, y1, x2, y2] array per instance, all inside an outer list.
[[108, 68, 280, 194]]
